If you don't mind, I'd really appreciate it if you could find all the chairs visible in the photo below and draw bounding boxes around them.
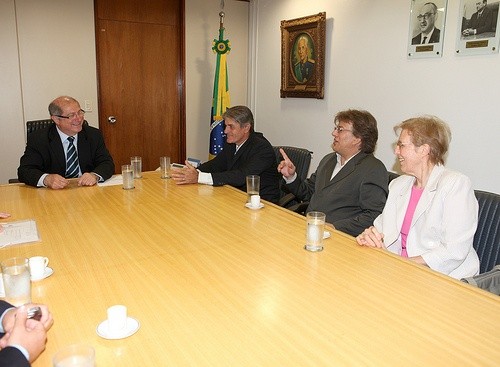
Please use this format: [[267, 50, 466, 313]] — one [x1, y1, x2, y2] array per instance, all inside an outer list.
[[463, 190, 500, 285], [288, 171, 401, 238], [26, 118, 88, 144], [271, 145, 315, 207]]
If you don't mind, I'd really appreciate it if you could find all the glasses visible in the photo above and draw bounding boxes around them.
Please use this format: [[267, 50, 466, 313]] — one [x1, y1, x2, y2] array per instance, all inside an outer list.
[[55, 109, 86, 120], [396, 141, 417, 149], [417, 13, 433, 21], [334, 127, 353, 133]]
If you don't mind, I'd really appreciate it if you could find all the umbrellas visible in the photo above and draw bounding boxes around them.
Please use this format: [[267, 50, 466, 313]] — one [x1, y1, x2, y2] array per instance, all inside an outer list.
[[209, 14, 231, 161]]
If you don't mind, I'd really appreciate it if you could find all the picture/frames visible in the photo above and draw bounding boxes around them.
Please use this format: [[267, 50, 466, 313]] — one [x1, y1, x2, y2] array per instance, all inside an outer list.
[[280, 11, 325, 99]]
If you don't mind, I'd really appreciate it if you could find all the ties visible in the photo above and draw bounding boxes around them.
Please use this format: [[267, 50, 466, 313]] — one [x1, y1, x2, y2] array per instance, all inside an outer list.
[[65, 136, 79, 178]]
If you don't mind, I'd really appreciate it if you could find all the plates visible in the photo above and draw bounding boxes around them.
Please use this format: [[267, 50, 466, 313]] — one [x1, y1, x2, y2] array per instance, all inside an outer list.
[[245, 203, 264, 208], [96, 317, 139, 340], [31, 265, 54, 280]]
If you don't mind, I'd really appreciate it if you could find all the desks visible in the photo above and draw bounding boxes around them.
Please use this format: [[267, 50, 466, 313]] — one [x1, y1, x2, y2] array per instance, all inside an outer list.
[[0, 167, 500, 366]]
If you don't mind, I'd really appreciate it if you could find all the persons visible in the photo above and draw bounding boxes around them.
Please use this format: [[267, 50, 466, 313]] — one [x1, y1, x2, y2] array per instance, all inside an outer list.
[[172, 105, 281, 204], [462, 0, 494, 36], [0, 299, 54, 367], [277, 107, 389, 237], [17, 95, 114, 190], [412, 2, 440, 44], [356, 115, 479, 279], [293, 37, 315, 82]]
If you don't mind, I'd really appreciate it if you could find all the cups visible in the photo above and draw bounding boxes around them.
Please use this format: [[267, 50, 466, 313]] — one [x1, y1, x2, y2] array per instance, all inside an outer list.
[[28, 257, 49, 276], [52, 345, 96, 367], [247, 175, 260, 204], [107, 305, 127, 331], [130, 157, 141, 178], [307, 211, 326, 251], [121, 165, 134, 189], [251, 195, 260, 207], [160, 157, 171, 179], [0, 257, 32, 308]]
